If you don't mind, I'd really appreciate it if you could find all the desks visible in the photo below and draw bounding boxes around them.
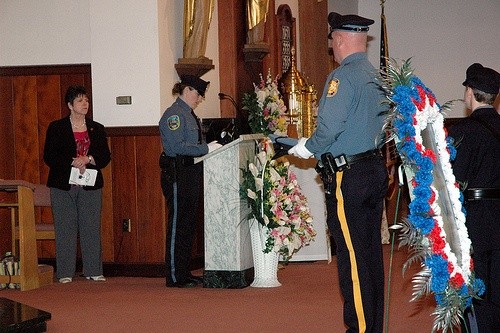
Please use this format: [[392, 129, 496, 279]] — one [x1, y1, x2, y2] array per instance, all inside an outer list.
[[0, 178, 54, 292]]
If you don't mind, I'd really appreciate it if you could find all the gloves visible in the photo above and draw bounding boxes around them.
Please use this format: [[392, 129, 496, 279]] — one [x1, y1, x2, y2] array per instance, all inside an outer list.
[[287, 137, 314, 160], [207, 140, 223, 154]]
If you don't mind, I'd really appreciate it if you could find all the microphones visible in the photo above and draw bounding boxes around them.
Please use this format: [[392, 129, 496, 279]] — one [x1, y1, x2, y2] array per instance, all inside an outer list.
[[219, 93, 253, 133]]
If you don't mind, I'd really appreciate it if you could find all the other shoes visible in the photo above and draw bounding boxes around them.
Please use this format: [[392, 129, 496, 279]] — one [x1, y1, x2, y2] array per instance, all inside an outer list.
[[59, 277, 73, 284], [86, 275, 106, 282]]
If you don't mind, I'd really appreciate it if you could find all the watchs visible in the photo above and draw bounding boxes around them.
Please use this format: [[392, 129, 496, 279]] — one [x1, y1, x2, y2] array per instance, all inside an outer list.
[[87, 155, 91, 162]]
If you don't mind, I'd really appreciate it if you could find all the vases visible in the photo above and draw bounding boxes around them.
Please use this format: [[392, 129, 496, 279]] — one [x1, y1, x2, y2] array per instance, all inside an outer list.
[[247, 216, 283, 288]]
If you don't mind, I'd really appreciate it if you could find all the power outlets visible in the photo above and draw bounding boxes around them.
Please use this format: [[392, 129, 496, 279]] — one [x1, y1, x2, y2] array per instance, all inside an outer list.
[[123, 219, 131, 233]]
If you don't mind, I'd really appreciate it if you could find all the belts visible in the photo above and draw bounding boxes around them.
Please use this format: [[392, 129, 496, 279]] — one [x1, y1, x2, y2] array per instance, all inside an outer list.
[[162, 151, 191, 159], [463, 188, 499, 201], [345, 149, 376, 163]]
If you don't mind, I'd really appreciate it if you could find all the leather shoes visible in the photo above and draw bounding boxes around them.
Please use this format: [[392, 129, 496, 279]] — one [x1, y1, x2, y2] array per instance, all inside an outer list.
[[186, 277, 203, 284], [166, 280, 197, 288]]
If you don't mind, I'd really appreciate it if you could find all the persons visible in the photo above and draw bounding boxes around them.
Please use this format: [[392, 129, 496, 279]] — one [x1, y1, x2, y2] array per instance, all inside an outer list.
[[287, 12, 390, 333], [450, 62, 500, 333], [44, 85, 112, 284], [158, 74, 217, 288]]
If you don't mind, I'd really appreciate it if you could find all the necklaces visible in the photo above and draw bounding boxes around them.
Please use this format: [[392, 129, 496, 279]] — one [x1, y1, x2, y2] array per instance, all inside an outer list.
[[70, 117, 85, 129]]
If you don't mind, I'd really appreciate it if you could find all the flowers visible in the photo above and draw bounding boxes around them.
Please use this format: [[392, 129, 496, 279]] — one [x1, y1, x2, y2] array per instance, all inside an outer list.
[[242, 67, 290, 138], [235, 138, 316, 265], [374, 55, 486, 332]]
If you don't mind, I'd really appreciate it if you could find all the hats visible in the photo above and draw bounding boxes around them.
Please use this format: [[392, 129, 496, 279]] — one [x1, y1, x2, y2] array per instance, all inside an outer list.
[[268, 134, 299, 160], [463, 63, 500, 94], [327, 12, 374, 39], [182, 75, 210, 97]]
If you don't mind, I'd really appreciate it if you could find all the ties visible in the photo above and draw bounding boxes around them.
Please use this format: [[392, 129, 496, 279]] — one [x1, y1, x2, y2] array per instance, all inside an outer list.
[[191, 109, 202, 145]]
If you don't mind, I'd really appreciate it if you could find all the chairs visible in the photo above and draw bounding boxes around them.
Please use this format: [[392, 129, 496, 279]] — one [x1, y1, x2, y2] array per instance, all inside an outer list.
[[12, 184, 56, 258]]
